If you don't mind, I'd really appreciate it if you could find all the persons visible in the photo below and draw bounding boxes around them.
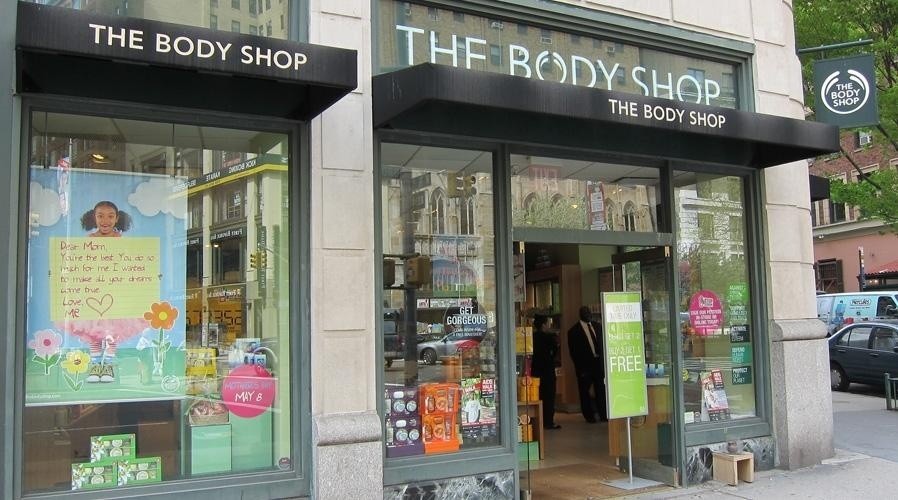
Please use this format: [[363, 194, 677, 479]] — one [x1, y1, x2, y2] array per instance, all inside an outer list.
[[79, 199, 132, 238], [528, 308, 563, 430], [463, 392, 482, 424], [703, 383, 720, 410], [566, 305, 610, 424], [833, 299, 847, 322]]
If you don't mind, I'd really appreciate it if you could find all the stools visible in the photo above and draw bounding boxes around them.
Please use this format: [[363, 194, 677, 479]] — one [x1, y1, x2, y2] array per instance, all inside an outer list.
[[712, 451, 754, 485]]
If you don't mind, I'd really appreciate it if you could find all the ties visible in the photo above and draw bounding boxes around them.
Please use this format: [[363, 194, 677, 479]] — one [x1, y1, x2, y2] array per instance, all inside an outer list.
[[587, 323, 601, 356]]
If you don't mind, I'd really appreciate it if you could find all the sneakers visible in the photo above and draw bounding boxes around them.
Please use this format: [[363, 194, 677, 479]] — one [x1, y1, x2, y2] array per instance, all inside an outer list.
[[100, 364, 115, 383], [87, 363, 100, 383]]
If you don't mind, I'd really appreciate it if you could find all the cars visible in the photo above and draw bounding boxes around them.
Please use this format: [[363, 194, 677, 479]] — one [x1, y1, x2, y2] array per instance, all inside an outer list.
[[382, 307, 491, 372], [828, 322, 898, 392]]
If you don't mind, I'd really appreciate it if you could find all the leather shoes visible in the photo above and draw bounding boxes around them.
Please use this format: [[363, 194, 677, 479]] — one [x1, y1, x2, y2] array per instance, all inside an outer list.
[[584, 414, 601, 425], [544, 423, 561, 429]]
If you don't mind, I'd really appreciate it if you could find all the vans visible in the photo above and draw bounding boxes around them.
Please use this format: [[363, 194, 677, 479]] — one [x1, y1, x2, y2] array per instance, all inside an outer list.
[[816, 291, 898, 320]]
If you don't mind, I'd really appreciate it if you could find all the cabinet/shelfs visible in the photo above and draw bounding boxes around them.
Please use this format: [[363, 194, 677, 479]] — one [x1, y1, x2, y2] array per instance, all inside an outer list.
[[518, 400, 545, 460], [525, 265, 581, 412]]
